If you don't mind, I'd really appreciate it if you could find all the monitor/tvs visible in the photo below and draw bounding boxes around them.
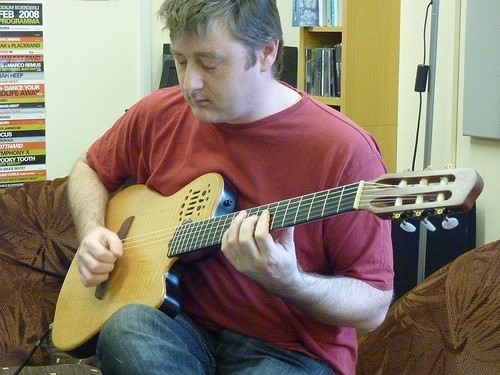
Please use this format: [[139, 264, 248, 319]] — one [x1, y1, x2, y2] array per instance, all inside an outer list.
[[158, 60, 180, 90]]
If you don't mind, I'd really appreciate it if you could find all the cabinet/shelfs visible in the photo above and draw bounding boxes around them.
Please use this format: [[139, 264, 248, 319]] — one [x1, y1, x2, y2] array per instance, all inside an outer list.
[[297, 0, 400, 173]]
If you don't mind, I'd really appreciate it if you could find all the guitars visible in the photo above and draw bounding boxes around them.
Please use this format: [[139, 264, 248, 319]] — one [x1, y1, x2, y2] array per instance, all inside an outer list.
[[51, 163, 484, 361]]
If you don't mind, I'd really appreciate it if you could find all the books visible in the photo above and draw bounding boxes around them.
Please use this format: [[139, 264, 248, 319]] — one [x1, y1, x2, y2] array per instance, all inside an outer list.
[[304, 41, 342, 97], [292, 0, 344, 27]]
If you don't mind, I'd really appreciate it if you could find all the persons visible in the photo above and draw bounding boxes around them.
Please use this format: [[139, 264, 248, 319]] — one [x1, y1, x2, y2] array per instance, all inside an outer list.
[[65, 0, 394, 375]]
